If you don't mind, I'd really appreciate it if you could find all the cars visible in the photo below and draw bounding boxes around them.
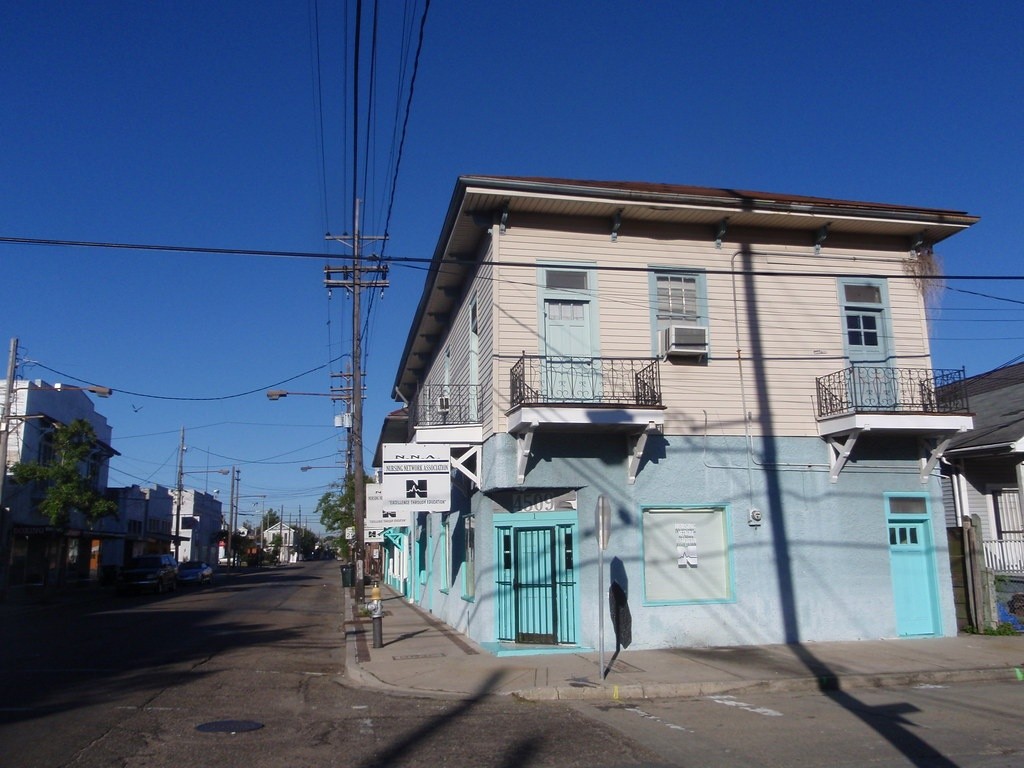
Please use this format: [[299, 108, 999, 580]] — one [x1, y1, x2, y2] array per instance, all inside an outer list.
[[178, 560, 213, 588]]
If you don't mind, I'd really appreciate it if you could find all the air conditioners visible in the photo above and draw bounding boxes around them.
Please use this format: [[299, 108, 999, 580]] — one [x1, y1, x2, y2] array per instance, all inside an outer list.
[[436, 397, 449, 415], [661, 325, 710, 357]]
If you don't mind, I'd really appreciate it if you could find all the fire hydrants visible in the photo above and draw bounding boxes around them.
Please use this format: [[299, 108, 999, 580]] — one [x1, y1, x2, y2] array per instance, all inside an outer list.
[[364, 581, 383, 649]]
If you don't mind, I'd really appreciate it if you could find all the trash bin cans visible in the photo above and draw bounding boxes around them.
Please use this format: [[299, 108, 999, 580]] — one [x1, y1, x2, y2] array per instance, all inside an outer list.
[[340, 564, 356, 588]]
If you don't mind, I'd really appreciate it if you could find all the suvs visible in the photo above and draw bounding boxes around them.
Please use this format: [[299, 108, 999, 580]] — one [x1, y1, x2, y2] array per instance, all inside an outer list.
[[122, 553, 180, 596]]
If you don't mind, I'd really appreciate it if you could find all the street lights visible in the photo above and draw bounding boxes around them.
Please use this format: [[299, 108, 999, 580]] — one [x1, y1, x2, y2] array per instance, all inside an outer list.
[[267, 388, 367, 601], [173, 424, 230, 563], [0, 337, 114, 506], [226, 465, 267, 570]]
